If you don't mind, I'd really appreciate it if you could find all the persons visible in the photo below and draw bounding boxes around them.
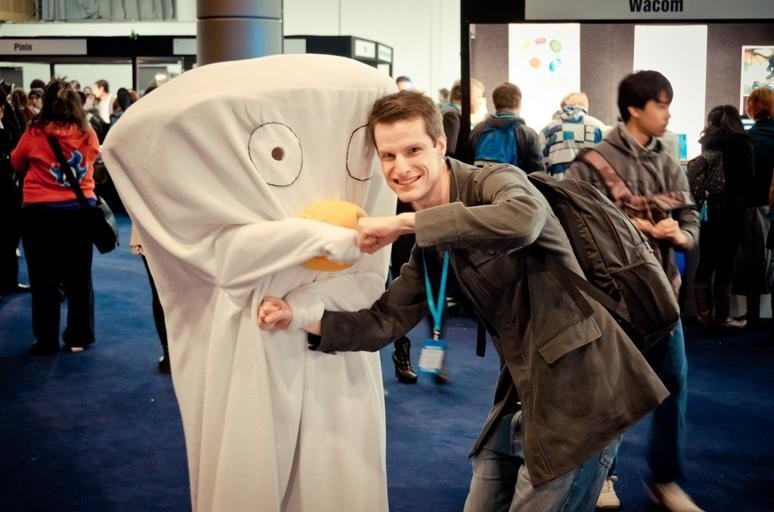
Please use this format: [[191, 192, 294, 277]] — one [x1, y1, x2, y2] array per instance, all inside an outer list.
[[257, 82, 674, 511], [563, 69, 704, 509], [1, 74, 171, 375], [377, 76, 772, 385]]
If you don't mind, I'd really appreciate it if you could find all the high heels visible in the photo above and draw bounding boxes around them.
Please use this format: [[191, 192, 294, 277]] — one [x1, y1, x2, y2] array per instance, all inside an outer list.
[[393, 337, 418, 384]]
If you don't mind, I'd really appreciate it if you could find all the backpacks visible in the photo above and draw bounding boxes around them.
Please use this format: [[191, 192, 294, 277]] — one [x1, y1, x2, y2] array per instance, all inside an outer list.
[[687, 150, 725, 208], [579, 147, 698, 296], [529, 170, 682, 351], [473, 121, 522, 166]]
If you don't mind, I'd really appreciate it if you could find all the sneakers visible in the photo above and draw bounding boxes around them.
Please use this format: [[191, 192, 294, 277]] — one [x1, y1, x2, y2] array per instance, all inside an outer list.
[[696, 310, 712, 322], [644, 480, 700, 511], [719, 317, 747, 328], [597, 476, 621, 510]]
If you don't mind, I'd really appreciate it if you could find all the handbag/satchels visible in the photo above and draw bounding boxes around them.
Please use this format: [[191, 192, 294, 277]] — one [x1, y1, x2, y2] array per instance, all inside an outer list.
[[93, 161, 107, 185], [94, 194, 119, 254]]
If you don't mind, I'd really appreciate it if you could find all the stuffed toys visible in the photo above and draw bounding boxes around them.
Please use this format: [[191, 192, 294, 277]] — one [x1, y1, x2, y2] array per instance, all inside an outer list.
[[100, 56, 398, 512]]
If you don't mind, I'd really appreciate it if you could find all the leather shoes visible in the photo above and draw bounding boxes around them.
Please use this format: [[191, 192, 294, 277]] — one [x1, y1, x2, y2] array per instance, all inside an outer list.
[[158, 341, 172, 374], [16, 283, 30, 293]]
